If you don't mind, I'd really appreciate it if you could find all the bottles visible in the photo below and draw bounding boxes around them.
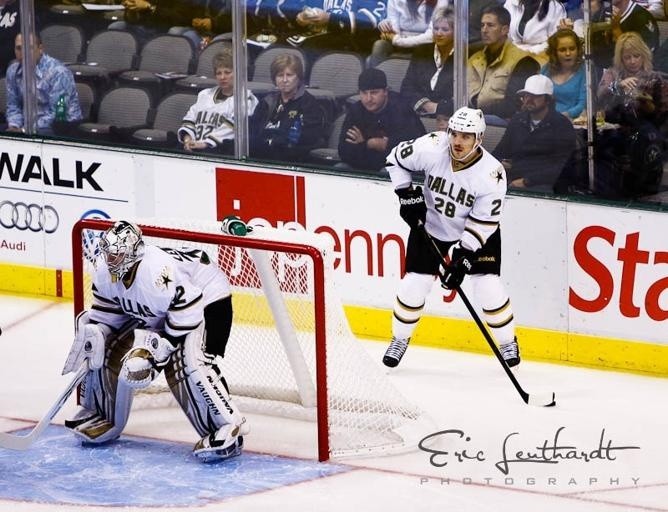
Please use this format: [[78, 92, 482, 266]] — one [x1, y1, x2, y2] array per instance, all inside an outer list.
[[288, 115, 305, 146], [54, 94, 67, 130]]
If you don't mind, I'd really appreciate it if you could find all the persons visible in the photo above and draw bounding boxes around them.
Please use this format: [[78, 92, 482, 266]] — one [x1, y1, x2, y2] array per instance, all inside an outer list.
[[1, 2, 667, 204], [62, 220, 251, 466], [382, 106, 521, 367]]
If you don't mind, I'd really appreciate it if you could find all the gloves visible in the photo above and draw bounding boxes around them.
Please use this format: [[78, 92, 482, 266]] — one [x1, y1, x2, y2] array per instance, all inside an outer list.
[[395, 185, 427, 230], [439, 240, 476, 290]]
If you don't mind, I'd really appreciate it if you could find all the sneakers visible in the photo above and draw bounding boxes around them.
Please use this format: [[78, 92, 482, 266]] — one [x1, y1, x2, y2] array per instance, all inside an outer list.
[[383, 337, 411, 367], [500, 336, 520, 367]]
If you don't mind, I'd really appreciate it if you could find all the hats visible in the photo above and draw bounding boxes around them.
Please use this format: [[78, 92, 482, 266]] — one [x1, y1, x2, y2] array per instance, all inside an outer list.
[[517, 74, 554, 97], [359, 69, 387, 90]]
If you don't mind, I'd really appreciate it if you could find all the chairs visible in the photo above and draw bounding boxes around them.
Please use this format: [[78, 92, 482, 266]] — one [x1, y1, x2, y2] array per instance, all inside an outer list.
[[653, 21, 668, 72], [481, 124, 506, 153], [0, 77, 7, 115]]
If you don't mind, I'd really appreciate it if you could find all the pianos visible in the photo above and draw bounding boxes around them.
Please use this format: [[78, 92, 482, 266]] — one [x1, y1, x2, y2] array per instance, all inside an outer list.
[[418, 218, 555, 407]]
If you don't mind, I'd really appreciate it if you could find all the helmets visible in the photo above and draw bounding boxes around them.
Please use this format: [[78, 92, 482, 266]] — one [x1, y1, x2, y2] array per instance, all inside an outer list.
[[447, 107, 486, 160], [100, 219, 145, 279]]
